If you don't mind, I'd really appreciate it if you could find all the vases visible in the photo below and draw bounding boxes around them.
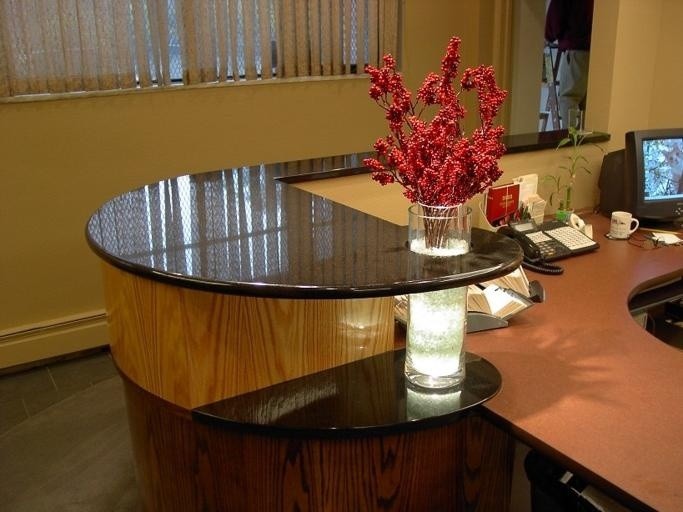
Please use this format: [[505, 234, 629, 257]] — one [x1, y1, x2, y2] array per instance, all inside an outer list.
[[405, 202, 472, 388]]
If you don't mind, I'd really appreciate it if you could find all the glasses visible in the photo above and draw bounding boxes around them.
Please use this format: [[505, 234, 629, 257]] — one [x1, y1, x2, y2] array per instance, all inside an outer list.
[[627, 235, 665, 250]]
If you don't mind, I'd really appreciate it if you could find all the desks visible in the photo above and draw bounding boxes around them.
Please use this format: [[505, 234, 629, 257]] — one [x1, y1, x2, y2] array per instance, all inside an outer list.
[[81, 120, 682, 511]]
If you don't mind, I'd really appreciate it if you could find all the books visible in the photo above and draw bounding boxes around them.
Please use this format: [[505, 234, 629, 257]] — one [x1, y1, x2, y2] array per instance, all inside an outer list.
[[487, 183, 519, 222]]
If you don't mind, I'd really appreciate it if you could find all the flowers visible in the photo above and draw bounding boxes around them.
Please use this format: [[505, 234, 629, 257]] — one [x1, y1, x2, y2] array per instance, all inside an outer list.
[[360, 34, 506, 248]]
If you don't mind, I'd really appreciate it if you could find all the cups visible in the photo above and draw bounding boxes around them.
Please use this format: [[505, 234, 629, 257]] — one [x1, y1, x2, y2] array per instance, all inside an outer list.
[[610, 211, 640, 239]]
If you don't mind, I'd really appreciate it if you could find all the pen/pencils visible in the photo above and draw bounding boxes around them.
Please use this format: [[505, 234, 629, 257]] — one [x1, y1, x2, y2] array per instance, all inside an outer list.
[[644, 235, 656, 242]]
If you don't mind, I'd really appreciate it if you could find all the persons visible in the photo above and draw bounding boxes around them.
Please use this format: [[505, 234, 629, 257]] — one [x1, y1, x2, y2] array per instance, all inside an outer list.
[[544, 0, 594, 130]]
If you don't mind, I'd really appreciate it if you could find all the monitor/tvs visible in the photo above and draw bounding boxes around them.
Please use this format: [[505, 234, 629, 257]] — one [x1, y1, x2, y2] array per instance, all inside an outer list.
[[598, 128, 683, 230]]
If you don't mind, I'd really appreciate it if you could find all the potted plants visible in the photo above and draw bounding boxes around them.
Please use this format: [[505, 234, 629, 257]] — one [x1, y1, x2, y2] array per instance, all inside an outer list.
[[539, 126, 610, 226]]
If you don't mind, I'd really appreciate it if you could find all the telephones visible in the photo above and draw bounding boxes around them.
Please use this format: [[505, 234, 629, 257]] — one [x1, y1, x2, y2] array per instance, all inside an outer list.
[[497, 218, 600, 265]]
